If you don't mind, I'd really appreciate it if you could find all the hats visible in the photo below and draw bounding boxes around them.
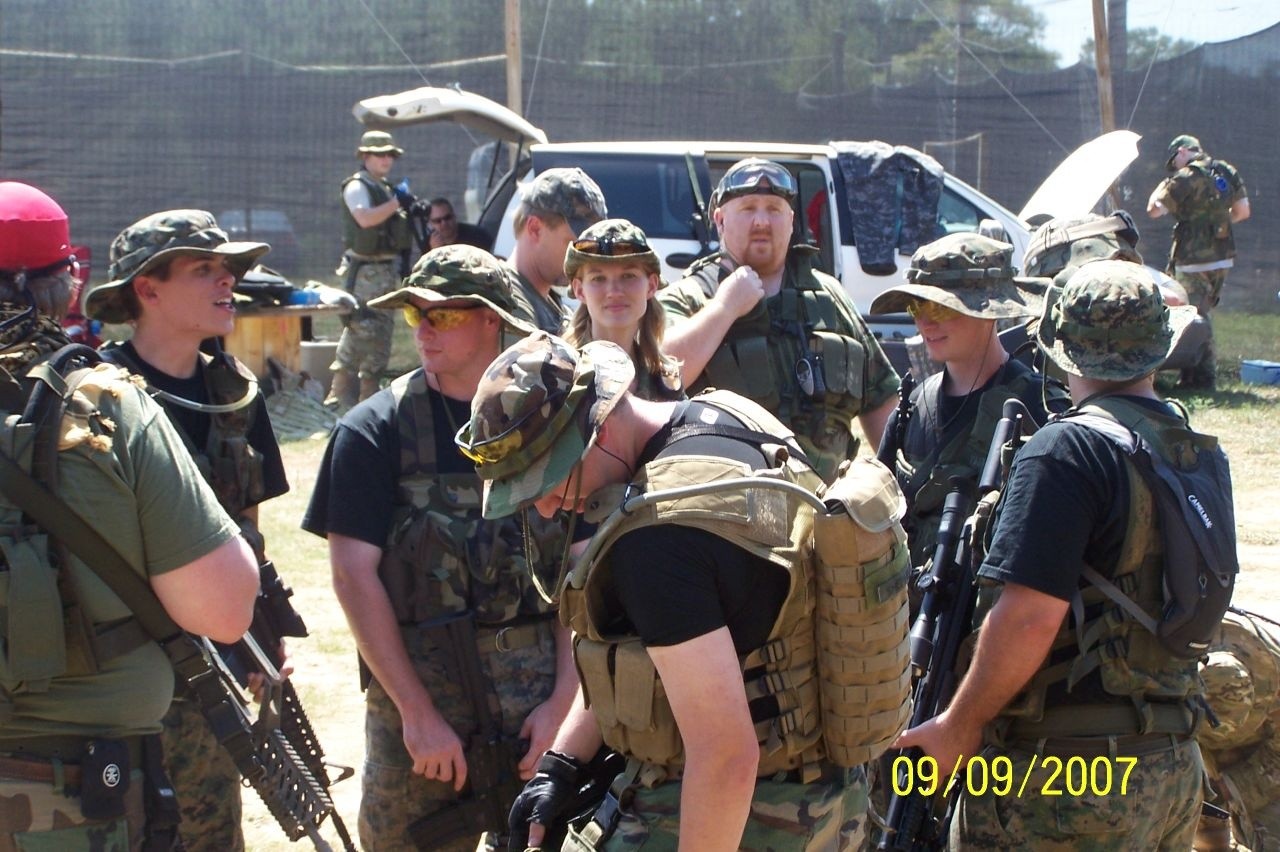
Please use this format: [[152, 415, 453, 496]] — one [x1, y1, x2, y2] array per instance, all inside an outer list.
[[1165, 134, 1200, 171], [366, 244, 538, 335], [563, 215, 661, 300], [1037, 259, 1199, 383], [464, 328, 637, 521], [0, 182, 74, 274], [869, 231, 1044, 320], [522, 165, 609, 236], [1196, 625, 1279, 747], [355, 131, 404, 158], [83, 209, 269, 324], [707, 157, 799, 221]]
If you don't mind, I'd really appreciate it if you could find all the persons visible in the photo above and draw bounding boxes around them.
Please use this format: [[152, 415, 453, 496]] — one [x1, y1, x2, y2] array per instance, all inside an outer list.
[[325, 130, 416, 414], [0, 181, 262, 852], [420, 197, 494, 253], [1147, 135, 1251, 396], [306, 156, 1280, 852], [84, 209, 296, 852]]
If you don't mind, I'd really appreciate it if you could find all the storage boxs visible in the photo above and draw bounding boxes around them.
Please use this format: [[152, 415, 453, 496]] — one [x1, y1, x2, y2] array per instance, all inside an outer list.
[[1240, 360, 1280, 388]]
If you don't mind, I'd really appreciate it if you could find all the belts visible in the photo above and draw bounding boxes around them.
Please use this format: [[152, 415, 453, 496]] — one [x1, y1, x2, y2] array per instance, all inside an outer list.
[[1017, 704, 1197, 740], [0, 758, 80, 784]]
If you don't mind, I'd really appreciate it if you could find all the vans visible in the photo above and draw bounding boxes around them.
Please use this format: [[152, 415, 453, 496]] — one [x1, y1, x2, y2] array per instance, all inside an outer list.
[[350, 83, 1143, 413]]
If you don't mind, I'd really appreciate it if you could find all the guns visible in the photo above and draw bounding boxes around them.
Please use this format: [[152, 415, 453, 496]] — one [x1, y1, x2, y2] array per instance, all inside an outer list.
[[228, 515, 356, 852], [876, 398, 1041, 852], [685, 153, 712, 261], [173, 633, 334, 852], [408, 736, 529, 852], [395, 189, 436, 254]]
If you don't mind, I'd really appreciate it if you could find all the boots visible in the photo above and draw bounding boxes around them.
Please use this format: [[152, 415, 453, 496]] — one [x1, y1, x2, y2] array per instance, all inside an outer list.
[[324, 370, 354, 416], [359, 378, 379, 403]]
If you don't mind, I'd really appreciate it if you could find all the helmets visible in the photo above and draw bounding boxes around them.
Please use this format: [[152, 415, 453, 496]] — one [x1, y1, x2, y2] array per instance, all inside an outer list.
[[1026, 214, 1143, 277]]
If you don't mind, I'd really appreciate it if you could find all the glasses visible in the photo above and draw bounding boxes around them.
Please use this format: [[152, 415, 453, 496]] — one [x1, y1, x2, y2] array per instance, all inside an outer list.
[[724, 161, 799, 199], [431, 212, 454, 224], [903, 297, 964, 323], [454, 389, 570, 467], [403, 301, 485, 332], [573, 239, 651, 257]]
